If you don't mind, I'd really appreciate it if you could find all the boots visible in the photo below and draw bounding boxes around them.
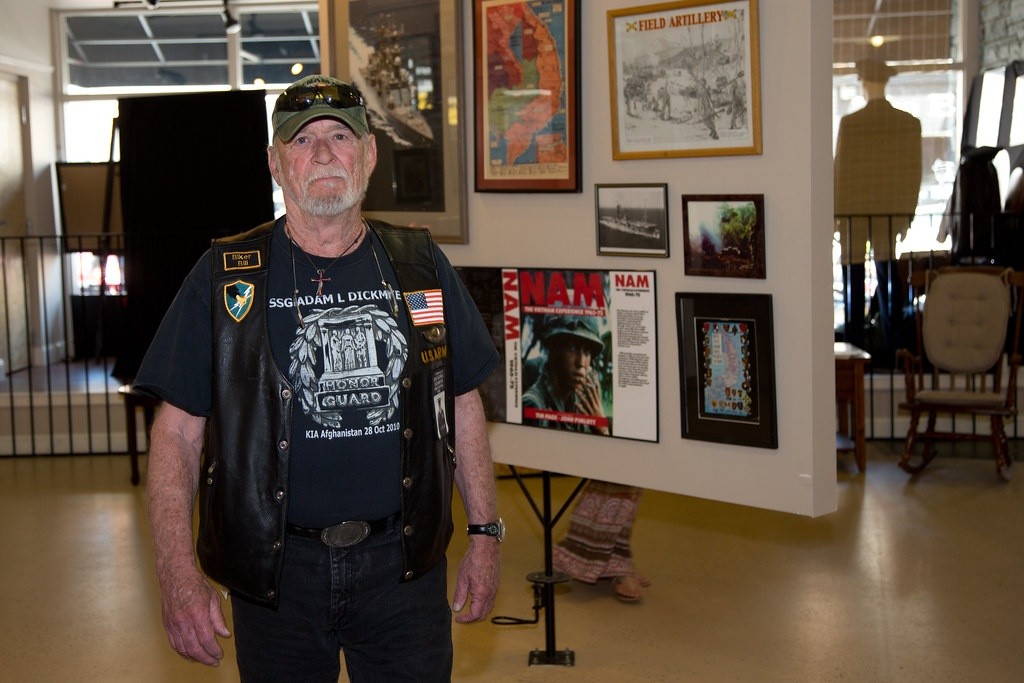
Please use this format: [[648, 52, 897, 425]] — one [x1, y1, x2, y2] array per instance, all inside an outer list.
[[834, 262, 866, 343], [870, 258, 903, 339]]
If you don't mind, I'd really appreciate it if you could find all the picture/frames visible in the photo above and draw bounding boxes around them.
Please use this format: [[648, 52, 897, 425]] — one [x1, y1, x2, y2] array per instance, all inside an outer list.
[[326, 0, 780, 449], [56, 161, 124, 253]]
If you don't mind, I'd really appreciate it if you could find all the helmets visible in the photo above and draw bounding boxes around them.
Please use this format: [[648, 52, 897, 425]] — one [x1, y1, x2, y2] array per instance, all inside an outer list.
[[542, 301, 605, 357]]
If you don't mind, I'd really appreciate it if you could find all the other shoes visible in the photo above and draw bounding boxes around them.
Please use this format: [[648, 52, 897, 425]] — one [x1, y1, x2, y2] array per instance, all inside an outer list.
[[607, 571, 642, 602], [634, 575, 650, 588]]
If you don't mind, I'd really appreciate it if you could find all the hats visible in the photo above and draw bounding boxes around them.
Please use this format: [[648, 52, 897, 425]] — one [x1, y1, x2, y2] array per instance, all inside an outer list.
[[851, 57, 899, 80], [271, 74, 370, 142]]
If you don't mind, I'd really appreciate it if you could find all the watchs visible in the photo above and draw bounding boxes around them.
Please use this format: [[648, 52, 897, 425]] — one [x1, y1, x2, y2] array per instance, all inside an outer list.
[[467, 518, 505, 545]]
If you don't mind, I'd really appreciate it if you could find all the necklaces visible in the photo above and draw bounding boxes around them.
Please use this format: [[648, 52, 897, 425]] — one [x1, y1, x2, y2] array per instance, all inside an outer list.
[[284, 223, 362, 295]]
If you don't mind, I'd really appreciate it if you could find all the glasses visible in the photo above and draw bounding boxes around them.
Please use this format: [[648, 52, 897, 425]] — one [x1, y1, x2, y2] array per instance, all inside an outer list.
[[559, 313, 599, 333], [272, 84, 365, 122]]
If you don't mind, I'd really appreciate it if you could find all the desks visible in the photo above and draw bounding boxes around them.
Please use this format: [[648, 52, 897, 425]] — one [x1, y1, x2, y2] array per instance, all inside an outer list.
[[833, 336, 874, 478]]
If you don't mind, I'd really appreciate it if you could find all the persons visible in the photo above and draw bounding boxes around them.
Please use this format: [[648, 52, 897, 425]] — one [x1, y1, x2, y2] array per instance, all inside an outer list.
[[554, 480, 651, 603], [526, 288, 611, 437], [130, 74, 507, 683], [830, 57, 922, 344]]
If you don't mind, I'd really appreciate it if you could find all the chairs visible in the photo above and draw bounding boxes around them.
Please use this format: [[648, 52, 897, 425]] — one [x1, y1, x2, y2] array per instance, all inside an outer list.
[[898, 267, 1024, 481]]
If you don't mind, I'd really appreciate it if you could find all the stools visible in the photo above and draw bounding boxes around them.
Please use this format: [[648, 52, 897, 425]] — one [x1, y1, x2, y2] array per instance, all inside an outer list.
[[119, 384, 164, 486]]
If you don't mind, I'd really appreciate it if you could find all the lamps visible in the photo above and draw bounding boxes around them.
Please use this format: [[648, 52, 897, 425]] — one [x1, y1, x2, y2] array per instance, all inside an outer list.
[[221, 0, 241, 35]]
